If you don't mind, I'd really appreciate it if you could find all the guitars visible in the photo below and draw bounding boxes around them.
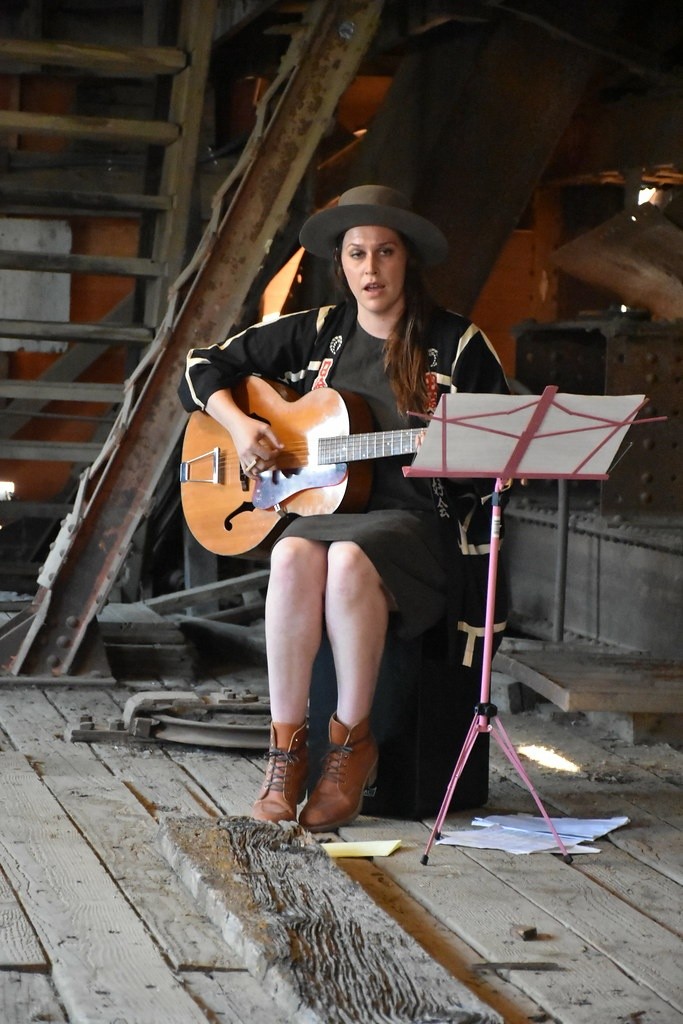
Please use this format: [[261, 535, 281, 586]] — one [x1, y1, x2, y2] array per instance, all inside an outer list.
[[176, 373, 428, 561]]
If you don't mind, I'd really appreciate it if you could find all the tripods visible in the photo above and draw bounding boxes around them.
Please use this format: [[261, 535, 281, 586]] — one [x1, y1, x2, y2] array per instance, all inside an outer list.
[[401, 392, 668, 866]]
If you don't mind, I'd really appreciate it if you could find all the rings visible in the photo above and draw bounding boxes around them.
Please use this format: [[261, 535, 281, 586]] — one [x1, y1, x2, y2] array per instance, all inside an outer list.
[[245, 456, 261, 472]]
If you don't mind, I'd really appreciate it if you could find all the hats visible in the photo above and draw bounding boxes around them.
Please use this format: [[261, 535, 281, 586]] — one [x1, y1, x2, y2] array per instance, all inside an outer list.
[[298, 185, 447, 270]]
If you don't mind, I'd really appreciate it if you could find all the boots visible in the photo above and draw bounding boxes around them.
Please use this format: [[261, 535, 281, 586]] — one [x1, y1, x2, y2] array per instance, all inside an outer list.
[[252, 717, 311, 823], [300, 711, 378, 832]]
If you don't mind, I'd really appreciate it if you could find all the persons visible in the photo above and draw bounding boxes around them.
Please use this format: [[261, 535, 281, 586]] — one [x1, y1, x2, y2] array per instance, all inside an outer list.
[[176, 184, 510, 833]]
